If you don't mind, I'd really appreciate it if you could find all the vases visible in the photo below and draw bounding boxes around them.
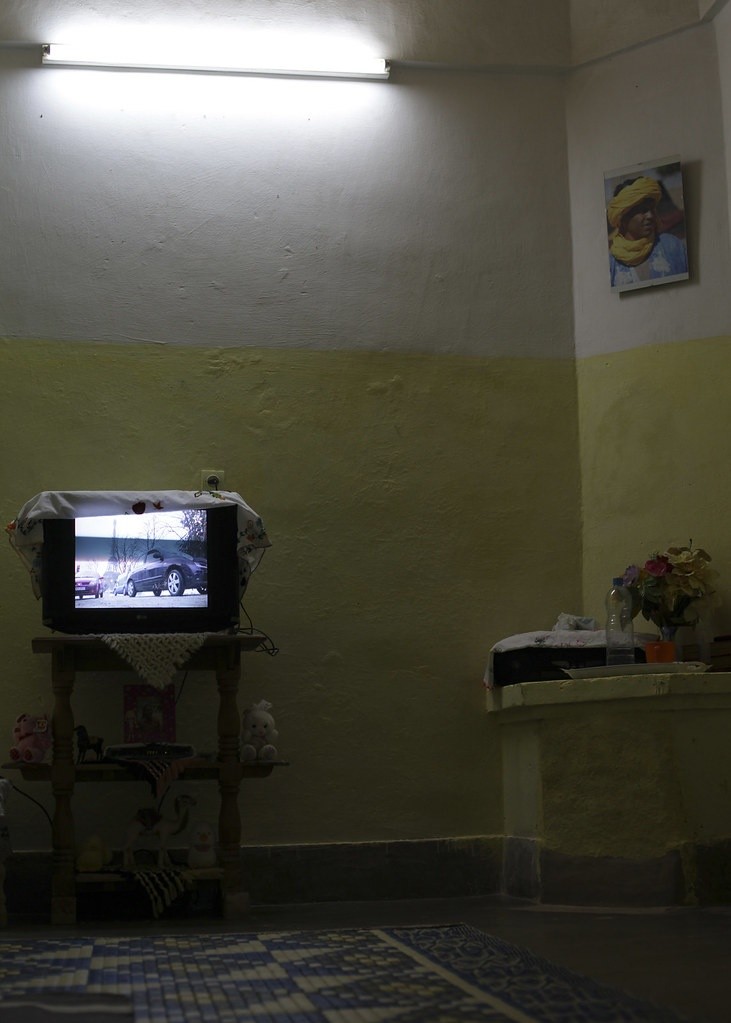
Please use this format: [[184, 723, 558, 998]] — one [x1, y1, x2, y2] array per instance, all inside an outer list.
[[660, 627, 693, 660]]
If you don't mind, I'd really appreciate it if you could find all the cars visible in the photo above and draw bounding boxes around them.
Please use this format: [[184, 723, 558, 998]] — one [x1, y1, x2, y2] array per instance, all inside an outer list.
[[113, 570, 130, 596], [126, 548, 209, 598], [75, 570, 104, 599]]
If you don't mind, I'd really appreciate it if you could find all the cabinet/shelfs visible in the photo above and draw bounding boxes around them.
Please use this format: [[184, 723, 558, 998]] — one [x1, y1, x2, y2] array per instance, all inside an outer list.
[[0, 632, 289, 926]]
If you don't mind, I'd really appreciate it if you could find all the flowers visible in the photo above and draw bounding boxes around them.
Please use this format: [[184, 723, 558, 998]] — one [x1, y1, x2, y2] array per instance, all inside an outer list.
[[612, 537, 720, 630]]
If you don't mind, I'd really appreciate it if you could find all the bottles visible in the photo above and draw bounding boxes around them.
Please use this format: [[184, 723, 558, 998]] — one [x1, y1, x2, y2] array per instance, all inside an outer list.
[[605, 576, 635, 666]]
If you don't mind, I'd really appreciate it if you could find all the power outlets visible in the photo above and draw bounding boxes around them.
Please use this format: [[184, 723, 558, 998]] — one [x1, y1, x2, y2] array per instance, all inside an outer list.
[[201, 469, 225, 490]]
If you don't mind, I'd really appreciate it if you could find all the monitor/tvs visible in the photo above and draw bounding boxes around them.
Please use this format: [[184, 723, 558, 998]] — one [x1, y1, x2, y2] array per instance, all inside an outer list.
[[44, 503, 241, 636]]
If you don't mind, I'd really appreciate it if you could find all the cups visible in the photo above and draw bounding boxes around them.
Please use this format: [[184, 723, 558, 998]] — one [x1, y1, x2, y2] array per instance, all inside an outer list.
[[644, 642, 676, 663]]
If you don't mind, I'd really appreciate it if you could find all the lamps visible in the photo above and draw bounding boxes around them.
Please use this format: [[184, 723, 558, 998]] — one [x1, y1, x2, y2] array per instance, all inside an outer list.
[[40, 43, 390, 80]]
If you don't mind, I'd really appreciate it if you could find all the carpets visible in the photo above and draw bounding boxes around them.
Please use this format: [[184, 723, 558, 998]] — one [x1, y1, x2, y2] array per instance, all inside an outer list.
[[0, 920, 688, 1023]]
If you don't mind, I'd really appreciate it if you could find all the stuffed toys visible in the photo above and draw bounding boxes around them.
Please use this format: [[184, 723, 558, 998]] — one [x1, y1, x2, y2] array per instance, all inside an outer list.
[[241, 700, 278, 761], [188, 820, 217, 870], [9, 713, 51, 764]]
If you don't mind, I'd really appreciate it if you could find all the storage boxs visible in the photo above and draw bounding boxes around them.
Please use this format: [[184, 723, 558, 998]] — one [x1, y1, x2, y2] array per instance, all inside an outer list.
[[492, 646, 647, 686]]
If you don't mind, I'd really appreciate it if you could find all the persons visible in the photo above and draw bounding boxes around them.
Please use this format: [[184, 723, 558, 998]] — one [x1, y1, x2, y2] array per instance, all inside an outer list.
[[610, 175, 689, 287]]
